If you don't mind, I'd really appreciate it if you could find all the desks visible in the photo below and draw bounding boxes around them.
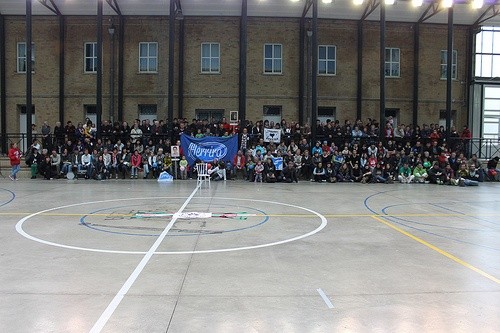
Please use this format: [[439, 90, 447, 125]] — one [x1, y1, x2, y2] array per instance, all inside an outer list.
[[165, 158, 180, 180]]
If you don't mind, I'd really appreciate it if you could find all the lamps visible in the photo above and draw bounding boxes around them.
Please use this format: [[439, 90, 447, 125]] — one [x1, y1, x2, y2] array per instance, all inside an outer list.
[[174, 9, 184, 23]]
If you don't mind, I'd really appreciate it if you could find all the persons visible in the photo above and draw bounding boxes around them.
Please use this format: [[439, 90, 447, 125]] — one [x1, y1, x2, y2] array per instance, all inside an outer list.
[[172, 147, 178, 156], [25, 116, 500, 188], [8, 142, 23, 181]]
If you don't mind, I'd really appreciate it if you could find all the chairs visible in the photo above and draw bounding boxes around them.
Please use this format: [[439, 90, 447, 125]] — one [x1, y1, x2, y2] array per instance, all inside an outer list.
[[195, 163, 211, 191]]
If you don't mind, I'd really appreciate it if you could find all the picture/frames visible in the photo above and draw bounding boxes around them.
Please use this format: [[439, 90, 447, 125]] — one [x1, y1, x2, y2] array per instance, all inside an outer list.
[[171, 146, 179, 157]]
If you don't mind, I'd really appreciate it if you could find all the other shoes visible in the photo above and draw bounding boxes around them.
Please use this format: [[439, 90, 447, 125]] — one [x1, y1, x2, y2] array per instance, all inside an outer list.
[[31, 176, 36, 179], [16, 178, 18, 181], [9, 175, 13, 181]]
